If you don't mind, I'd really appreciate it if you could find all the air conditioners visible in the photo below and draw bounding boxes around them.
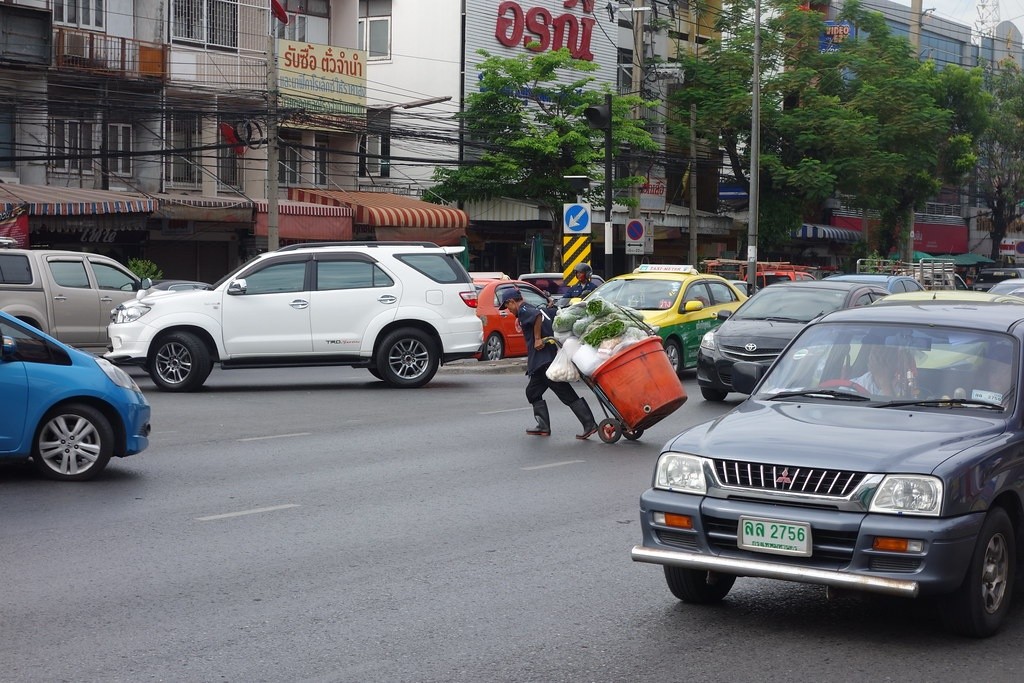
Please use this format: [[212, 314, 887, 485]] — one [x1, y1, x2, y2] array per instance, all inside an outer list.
[[56, 32, 90, 59]]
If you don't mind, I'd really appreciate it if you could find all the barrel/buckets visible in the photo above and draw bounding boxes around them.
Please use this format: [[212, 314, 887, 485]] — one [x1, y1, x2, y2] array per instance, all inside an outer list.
[[592, 336, 688, 431]]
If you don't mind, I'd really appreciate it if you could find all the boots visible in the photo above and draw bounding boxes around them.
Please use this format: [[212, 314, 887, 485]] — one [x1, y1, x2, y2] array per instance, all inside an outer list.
[[569, 397, 599, 439], [525, 399, 551, 436]]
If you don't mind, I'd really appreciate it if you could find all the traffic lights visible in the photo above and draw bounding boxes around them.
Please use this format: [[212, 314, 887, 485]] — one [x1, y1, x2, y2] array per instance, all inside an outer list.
[[583, 105, 611, 129]]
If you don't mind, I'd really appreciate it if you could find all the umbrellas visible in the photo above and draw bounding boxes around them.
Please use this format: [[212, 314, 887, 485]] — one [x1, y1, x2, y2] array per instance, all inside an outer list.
[[890, 250, 996, 273]]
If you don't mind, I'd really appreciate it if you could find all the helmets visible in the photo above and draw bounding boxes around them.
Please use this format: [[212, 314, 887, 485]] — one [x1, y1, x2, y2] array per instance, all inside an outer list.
[[574, 263, 592, 283]]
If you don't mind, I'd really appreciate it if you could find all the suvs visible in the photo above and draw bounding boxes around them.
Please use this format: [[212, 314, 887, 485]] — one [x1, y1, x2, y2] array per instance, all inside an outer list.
[[99, 241, 606, 393]]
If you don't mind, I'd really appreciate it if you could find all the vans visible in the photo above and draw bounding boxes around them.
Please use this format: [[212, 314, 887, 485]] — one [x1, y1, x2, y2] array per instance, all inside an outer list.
[[0, 248, 153, 348]]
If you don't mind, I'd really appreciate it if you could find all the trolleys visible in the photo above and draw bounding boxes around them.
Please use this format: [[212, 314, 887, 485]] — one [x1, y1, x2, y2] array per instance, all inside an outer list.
[[543, 337, 666, 443]]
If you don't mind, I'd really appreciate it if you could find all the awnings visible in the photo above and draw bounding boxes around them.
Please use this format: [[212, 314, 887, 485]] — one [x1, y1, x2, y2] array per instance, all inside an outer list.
[[0, 182, 159, 233], [250, 198, 354, 240], [288, 187, 470, 246], [786, 223, 864, 244], [138, 192, 258, 223]]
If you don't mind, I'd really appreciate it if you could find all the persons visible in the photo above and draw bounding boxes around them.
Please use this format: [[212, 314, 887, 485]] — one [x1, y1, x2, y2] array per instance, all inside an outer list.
[[838, 338, 913, 398], [562, 263, 598, 300], [498, 289, 598, 439]]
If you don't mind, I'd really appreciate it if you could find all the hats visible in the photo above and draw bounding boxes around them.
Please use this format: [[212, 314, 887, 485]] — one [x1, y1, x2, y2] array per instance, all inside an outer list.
[[498, 288, 519, 310]]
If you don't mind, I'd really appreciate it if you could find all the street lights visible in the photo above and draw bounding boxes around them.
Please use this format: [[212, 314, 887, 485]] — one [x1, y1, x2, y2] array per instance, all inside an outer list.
[[356, 95, 452, 191]]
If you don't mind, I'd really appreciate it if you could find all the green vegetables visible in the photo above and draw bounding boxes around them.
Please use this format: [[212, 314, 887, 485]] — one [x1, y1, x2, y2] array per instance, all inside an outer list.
[[552, 297, 652, 347]]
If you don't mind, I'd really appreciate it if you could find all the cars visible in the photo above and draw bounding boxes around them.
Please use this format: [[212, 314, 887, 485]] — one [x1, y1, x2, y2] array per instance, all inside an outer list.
[[0, 309, 152, 481], [569, 264, 750, 376], [631, 258, 1024, 638]]
